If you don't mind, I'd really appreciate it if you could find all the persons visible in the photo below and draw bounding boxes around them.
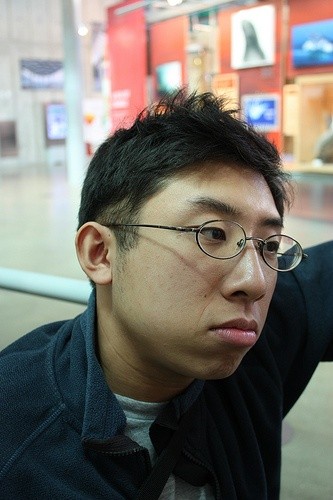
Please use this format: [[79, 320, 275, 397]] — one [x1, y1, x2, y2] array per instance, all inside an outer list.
[[1, 85, 333, 500]]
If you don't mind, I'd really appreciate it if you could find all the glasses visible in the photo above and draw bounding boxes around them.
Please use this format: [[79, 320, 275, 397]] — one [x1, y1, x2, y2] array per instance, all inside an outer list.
[[101, 219, 308, 272]]
